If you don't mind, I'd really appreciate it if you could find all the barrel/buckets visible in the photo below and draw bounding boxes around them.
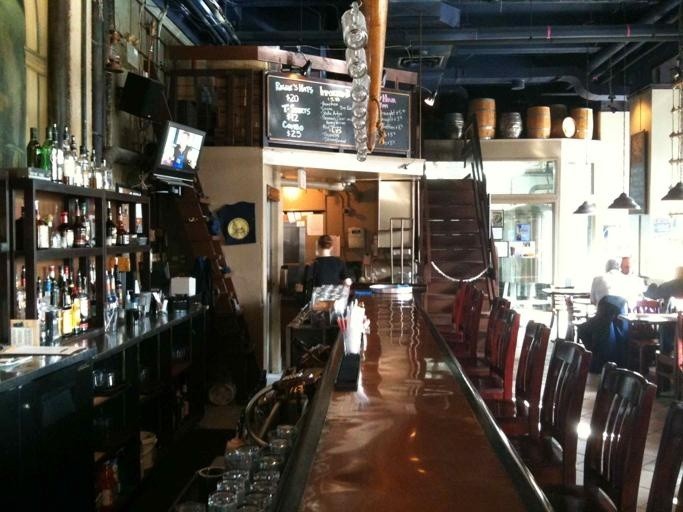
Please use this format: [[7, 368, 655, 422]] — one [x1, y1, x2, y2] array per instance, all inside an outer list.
[[553, 116, 577, 138], [553, 103, 569, 119], [571, 106, 595, 138], [525, 106, 554, 138], [471, 98, 496, 139], [446, 112, 465, 140]]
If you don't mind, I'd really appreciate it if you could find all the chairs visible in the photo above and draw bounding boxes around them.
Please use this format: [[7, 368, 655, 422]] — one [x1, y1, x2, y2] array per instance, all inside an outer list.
[[455, 298, 511, 377], [482, 320, 551, 437], [542, 362, 658, 510], [646, 400, 682, 510], [503, 339, 593, 490], [547, 278, 683, 398], [435, 280, 485, 358]]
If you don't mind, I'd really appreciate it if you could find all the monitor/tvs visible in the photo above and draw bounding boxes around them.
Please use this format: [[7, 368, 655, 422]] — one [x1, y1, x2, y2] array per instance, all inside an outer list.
[[153, 120, 208, 179], [514, 222, 532, 241]]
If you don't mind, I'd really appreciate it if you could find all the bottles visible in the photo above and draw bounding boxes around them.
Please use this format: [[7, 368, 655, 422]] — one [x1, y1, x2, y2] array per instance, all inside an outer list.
[[207, 423, 296, 511], [466, 98, 496, 140], [525, 103, 595, 139], [115, 207, 129, 244], [105, 200, 119, 244], [13, 256, 122, 341], [107, 372, 114, 387], [35, 198, 93, 250], [442, 112, 463, 140], [281, 265, 288, 289], [97, 461, 118, 489], [25, 122, 110, 190]]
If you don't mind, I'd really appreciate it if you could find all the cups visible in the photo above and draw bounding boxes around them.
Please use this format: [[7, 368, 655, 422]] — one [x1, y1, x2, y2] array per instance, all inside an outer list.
[[125, 308, 135, 324], [343, 330, 361, 357], [340, 1, 369, 161], [198, 466, 227, 502], [102, 489, 113, 506], [124, 324, 135, 338]]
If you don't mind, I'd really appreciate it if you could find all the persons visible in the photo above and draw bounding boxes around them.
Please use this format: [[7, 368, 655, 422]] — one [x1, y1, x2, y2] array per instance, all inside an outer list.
[[620, 255, 631, 274], [307, 235, 347, 294], [174, 132, 199, 169], [590, 259, 644, 313]]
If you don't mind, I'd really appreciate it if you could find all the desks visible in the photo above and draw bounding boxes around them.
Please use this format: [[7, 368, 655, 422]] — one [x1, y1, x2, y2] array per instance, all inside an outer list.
[[276, 296, 554, 511]]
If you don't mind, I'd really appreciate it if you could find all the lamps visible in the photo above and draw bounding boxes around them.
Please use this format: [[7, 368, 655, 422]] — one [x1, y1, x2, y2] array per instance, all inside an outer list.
[[660, 99, 683, 202], [571, 99, 601, 217], [410, 82, 438, 109], [379, 69, 389, 89], [294, 45, 313, 79], [607, 100, 641, 212]]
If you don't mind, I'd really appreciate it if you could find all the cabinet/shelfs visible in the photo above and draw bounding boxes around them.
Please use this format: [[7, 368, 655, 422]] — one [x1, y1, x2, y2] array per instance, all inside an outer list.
[[91, 308, 211, 510], [0, 167, 16, 348], [0, 357, 91, 511], [10, 177, 153, 348]]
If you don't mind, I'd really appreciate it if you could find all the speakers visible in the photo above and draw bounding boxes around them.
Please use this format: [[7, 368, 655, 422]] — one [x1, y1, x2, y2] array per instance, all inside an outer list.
[[117, 72, 167, 121]]
